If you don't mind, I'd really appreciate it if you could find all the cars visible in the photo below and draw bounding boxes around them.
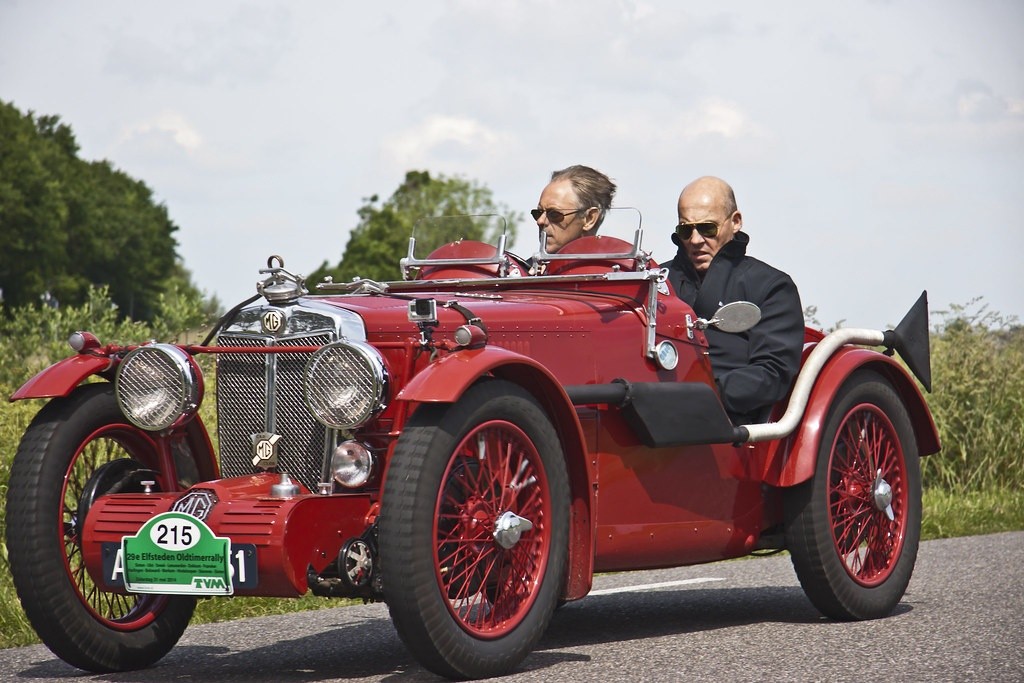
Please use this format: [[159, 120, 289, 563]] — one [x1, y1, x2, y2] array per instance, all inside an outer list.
[[8, 207, 944, 679]]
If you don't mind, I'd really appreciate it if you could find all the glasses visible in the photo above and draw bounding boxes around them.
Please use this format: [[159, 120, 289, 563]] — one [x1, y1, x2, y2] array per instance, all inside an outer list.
[[531, 209, 579, 223], [676, 211, 734, 240]]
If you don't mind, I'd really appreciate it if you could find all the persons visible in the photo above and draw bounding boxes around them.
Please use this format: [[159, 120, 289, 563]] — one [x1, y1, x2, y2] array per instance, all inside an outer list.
[[526, 165, 617, 266], [657, 176, 805, 426]]
